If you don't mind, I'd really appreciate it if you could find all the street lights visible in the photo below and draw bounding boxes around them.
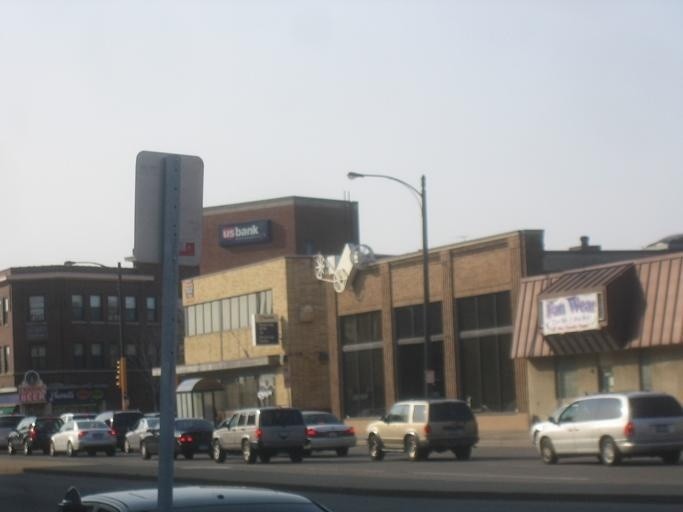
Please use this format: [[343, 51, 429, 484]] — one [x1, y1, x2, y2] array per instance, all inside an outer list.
[[63, 260, 128, 409], [347, 172, 433, 397]]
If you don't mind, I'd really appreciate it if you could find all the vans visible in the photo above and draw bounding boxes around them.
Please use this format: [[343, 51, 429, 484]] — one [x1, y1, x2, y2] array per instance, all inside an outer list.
[[0, 414, 25, 449]]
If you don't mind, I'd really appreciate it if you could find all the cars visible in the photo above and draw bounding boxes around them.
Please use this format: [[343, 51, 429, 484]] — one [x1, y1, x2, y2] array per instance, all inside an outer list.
[[7, 409, 215, 458], [529, 392, 682, 465], [57, 484, 330, 512], [301, 411, 357, 458], [365, 399, 480, 462]]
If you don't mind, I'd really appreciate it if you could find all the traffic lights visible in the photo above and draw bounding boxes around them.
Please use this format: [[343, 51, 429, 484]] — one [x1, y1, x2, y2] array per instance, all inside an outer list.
[[114, 359, 120, 388]]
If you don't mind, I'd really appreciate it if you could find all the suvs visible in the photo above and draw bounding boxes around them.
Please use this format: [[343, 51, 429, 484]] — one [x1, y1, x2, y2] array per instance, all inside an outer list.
[[209, 408, 311, 464]]
